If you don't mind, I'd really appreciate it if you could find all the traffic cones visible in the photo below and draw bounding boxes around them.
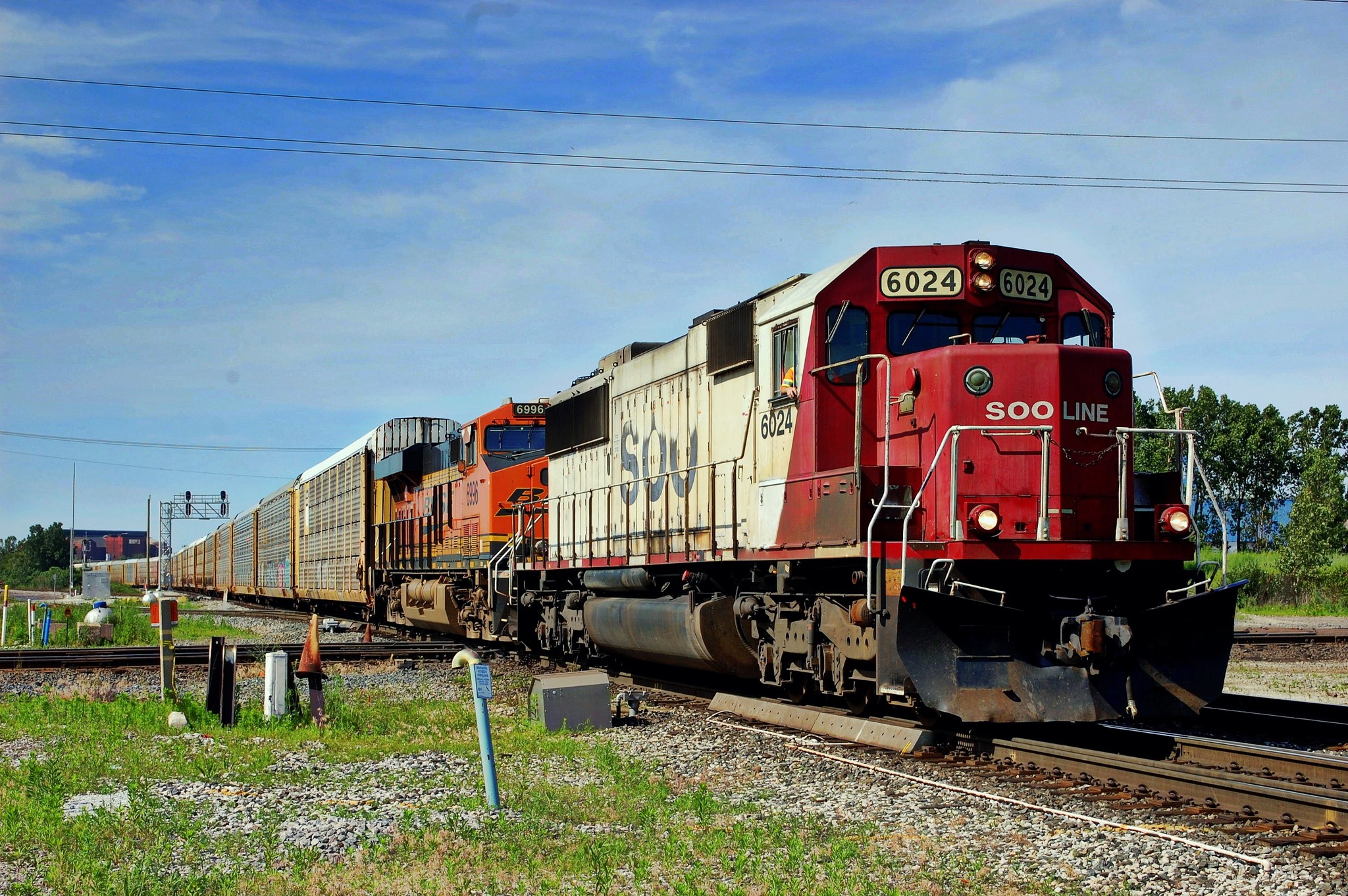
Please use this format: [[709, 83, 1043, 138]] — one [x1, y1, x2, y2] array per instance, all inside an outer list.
[[295, 613, 330, 679], [362, 622, 372, 642]]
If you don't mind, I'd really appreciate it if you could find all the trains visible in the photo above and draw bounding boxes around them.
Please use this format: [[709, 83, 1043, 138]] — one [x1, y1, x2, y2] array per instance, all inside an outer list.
[[69, 237, 1248, 728]]
